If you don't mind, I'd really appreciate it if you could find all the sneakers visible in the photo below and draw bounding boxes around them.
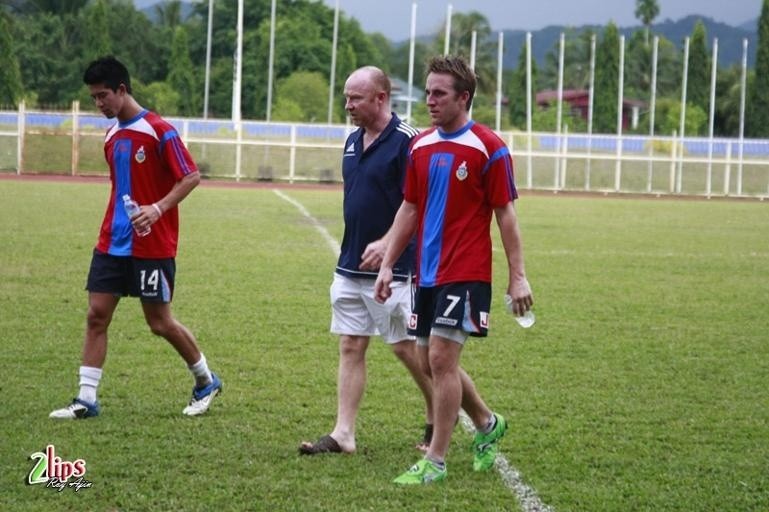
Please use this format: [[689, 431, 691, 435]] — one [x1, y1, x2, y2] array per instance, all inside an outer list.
[[179, 372, 221, 417], [469, 413, 509, 474], [49, 398, 103, 420], [392, 458, 449, 487]]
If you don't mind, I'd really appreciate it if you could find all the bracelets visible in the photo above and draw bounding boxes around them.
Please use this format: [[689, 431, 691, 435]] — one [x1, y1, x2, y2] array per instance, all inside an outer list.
[[151, 203, 163, 219]]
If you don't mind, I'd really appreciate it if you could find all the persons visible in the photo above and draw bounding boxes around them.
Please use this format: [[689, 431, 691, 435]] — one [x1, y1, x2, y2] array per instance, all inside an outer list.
[[373, 53, 535, 488], [298, 63, 463, 460], [47, 54, 225, 420]]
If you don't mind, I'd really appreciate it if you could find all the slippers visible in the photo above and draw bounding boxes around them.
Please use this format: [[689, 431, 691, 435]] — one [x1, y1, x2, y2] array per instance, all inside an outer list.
[[298, 434, 348, 455], [422, 411, 460, 455]]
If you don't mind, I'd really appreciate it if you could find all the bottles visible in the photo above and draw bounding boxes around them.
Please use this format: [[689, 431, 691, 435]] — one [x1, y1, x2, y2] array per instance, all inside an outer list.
[[122, 194, 152, 238], [502, 293, 537, 329]]
